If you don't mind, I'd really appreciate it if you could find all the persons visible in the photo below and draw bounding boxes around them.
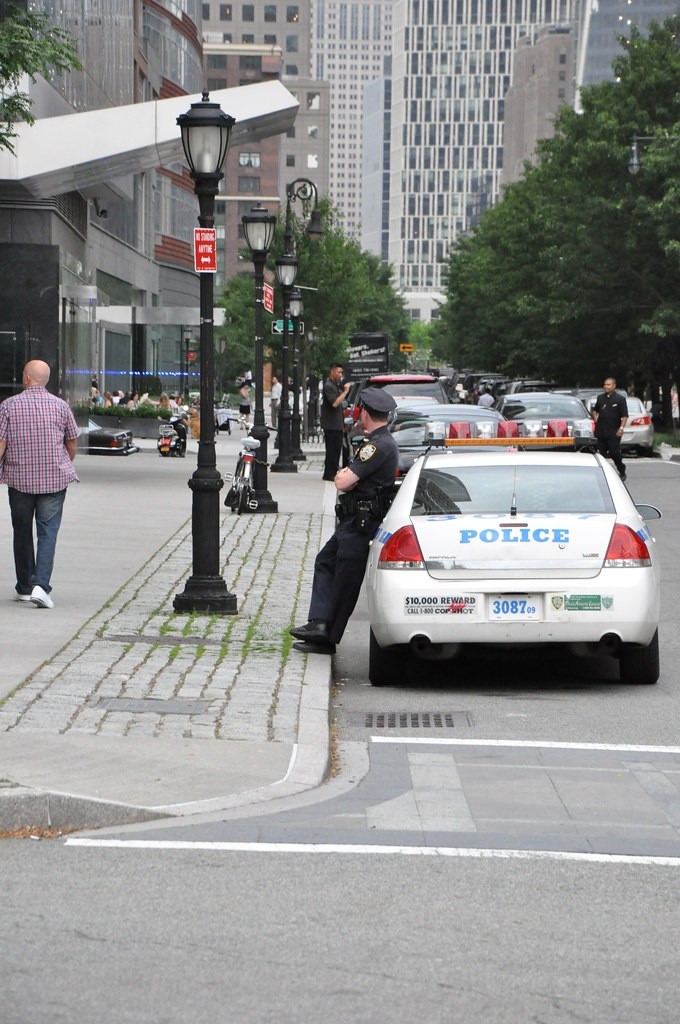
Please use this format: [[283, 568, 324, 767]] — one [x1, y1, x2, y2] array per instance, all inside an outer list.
[[592, 378, 629, 481], [320, 361, 352, 482], [269, 377, 283, 429], [0, 360, 81, 609], [671, 385, 680, 431], [90, 375, 201, 421], [288, 386, 400, 655], [239, 382, 251, 429], [477, 388, 495, 407]]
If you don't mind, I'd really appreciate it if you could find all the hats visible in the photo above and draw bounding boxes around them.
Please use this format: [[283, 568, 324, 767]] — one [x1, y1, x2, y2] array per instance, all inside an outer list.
[[360, 387, 398, 418]]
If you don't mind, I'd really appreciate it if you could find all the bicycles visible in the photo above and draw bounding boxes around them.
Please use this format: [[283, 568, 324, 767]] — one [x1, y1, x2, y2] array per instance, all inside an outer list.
[[224, 417, 279, 515]]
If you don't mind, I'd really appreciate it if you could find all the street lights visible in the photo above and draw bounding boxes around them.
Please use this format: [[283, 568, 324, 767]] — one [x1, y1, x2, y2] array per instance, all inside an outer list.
[[236, 199, 282, 514], [170, 86, 238, 614], [287, 286, 308, 462], [269, 247, 302, 473], [274, 178, 327, 450]]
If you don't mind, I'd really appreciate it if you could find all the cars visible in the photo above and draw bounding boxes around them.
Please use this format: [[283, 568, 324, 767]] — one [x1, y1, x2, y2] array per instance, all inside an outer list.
[[487, 392, 598, 454], [582, 396, 656, 457], [71, 413, 141, 457], [364, 418, 665, 686], [340, 367, 629, 468], [388, 404, 525, 510]]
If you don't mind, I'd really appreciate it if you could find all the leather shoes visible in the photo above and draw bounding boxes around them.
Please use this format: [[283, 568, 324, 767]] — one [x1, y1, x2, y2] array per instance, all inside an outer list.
[[289, 616, 328, 643], [292, 634, 340, 655]]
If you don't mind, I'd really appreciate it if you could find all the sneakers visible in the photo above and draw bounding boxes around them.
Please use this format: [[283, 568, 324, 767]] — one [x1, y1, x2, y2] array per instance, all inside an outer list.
[[17, 590, 30, 603], [30, 583, 54, 609]]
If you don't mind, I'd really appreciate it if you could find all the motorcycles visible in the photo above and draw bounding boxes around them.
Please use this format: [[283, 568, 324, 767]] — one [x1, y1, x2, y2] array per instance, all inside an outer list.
[[156, 412, 189, 457]]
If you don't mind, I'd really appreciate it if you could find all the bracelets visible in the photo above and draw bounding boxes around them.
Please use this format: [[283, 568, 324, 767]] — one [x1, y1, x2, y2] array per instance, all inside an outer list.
[[620, 426, 623, 430]]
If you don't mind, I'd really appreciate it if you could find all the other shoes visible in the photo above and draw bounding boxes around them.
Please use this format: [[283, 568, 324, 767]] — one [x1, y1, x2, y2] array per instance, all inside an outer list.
[[620, 466, 627, 482]]
